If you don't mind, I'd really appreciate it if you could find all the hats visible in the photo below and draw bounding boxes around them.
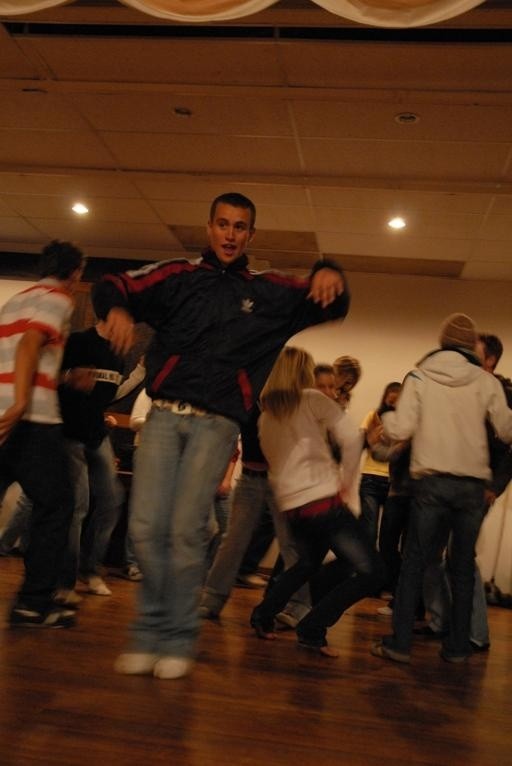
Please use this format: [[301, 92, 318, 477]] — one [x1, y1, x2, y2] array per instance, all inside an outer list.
[[439, 313, 478, 352]]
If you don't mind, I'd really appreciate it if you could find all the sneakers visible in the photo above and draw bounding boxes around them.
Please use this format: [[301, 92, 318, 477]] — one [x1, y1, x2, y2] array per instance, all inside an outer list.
[[443, 652, 468, 666], [53, 586, 84, 610], [112, 558, 142, 581], [114, 648, 157, 675], [369, 641, 412, 667], [7, 596, 78, 632], [151, 653, 191, 681]]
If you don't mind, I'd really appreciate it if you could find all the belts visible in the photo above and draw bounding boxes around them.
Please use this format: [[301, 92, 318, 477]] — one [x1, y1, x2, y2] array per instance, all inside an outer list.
[[152, 397, 207, 417]]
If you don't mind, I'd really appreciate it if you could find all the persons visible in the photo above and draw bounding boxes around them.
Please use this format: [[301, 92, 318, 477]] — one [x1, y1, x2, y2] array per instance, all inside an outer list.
[[357, 379, 409, 617], [203, 436, 244, 573], [249, 345, 393, 653], [0, 347, 152, 557], [55, 277, 131, 608], [0, 235, 78, 631], [335, 351, 363, 416], [368, 312, 512, 662], [196, 397, 316, 630], [121, 386, 154, 586], [260, 363, 340, 608], [87, 191, 350, 683], [411, 330, 512, 651], [367, 369, 430, 624]]
